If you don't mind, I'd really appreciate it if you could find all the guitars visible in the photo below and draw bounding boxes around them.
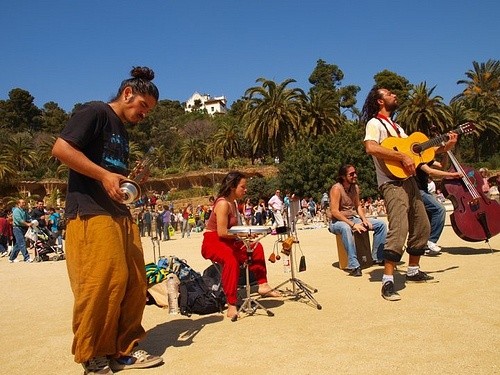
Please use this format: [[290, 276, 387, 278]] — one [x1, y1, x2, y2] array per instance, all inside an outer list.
[[378, 120, 477, 180]]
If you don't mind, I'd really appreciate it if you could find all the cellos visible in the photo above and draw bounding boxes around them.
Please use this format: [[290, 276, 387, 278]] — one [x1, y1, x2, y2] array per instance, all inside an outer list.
[[429, 124, 500, 254]]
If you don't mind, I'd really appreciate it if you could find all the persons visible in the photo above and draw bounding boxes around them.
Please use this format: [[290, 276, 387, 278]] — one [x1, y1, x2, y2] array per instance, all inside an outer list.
[[130, 189, 388, 241], [52, 65, 164, 375], [328, 164, 388, 275], [363, 86, 458, 300], [0, 199, 68, 263], [415, 159, 464, 256], [201, 171, 284, 318], [427, 168, 500, 202]]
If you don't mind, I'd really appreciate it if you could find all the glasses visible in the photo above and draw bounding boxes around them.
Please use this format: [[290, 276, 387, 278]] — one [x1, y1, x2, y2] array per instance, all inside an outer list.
[[345, 171, 356, 176]]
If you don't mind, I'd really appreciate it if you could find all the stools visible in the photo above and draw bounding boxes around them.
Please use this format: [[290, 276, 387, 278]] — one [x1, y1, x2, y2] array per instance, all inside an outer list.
[[335, 229, 373, 271], [208, 258, 259, 316]]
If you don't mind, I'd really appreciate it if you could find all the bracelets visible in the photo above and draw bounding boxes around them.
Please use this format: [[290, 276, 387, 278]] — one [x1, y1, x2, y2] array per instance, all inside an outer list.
[[351, 223, 355, 228]]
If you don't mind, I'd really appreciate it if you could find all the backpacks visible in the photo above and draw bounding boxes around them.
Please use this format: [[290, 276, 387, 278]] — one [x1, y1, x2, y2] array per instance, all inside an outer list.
[[53, 215, 62, 230], [140, 255, 260, 316]]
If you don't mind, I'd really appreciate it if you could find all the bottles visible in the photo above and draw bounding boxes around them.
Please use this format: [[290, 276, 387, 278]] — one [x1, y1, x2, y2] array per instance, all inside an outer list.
[[283, 254, 290, 273], [166, 277, 178, 314]]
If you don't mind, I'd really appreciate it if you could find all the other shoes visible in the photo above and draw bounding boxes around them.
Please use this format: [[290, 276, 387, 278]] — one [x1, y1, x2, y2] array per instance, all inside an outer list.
[[376, 259, 387, 266], [351, 267, 362, 275], [9, 258, 19, 263], [1, 251, 8, 257], [25, 258, 33, 263], [427, 240, 441, 252]]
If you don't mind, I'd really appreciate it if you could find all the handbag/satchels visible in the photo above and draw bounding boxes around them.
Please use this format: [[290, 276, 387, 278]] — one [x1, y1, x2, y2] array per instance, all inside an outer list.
[[188, 214, 195, 223], [24, 225, 36, 243]]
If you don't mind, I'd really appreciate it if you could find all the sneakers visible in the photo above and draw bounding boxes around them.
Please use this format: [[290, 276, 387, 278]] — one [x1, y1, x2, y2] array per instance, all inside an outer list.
[[108, 349, 163, 369], [81, 354, 113, 375], [406, 269, 438, 282], [382, 280, 401, 300]]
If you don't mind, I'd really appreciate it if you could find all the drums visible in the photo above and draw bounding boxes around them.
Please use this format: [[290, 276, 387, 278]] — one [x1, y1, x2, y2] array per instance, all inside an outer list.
[[228, 225, 272, 236]]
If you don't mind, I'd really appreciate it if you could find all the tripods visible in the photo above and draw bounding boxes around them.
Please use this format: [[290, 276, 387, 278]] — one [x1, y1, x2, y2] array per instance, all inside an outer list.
[[231, 223, 322, 322]]
